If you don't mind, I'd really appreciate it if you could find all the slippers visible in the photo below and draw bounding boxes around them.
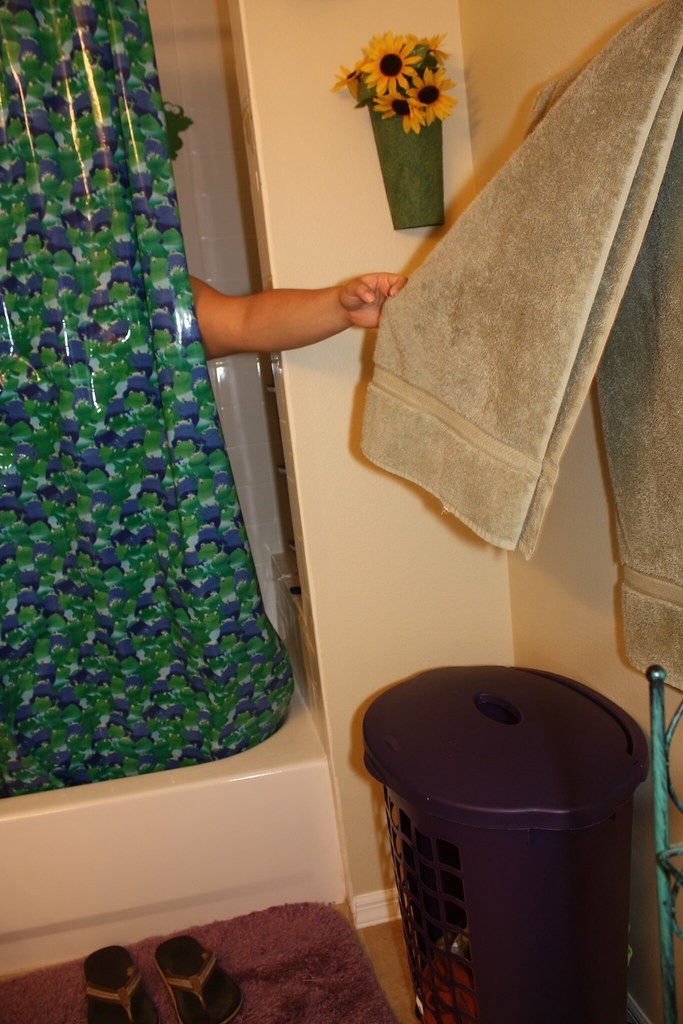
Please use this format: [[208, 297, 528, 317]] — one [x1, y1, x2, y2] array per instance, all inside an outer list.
[[152, 935, 242, 1024], [83, 945, 158, 1024]]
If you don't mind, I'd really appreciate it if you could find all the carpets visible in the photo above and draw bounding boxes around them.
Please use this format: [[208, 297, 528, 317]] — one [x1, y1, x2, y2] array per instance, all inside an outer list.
[[0, 902, 400, 1024]]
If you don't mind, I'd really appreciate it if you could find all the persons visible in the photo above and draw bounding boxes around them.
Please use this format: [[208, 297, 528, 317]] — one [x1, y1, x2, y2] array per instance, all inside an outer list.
[[185, 271, 408, 363]]
